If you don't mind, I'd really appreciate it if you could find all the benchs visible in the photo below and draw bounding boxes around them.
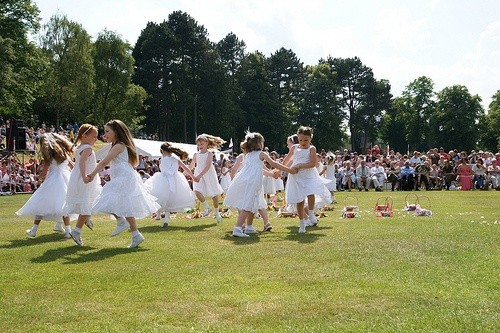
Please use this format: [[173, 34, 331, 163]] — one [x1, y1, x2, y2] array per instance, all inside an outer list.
[[351, 176, 401, 192]]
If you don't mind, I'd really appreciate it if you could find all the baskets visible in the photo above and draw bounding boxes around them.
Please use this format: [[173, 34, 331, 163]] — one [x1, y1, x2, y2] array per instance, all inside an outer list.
[[272, 193, 286, 211], [314, 211, 324, 218], [281, 203, 295, 217], [342, 193, 431, 218], [324, 200, 334, 211]]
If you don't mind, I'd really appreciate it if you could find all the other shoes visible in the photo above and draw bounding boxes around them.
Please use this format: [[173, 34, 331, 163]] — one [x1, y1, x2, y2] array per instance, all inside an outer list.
[[155, 216, 161, 220], [218, 217, 223, 223], [331, 198, 336, 204], [26, 230, 36, 236], [85, 220, 94, 230], [245, 228, 258, 233], [233, 230, 250, 238], [64, 233, 71, 239], [110, 220, 130, 237], [299, 210, 319, 233], [337, 186, 443, 192], [202, 208, 212, 217], [69, 232, 83, 246], [263, 222, 272, 232], [162, 220, 168, 228], [53, 226, 65, 233], [128, 232, 145, 250]]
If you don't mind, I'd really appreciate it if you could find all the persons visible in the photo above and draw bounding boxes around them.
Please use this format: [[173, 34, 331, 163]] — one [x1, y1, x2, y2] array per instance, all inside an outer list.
[[272, 132, 318, 228], [63, 123, 103, 245], [276, 124, 333, 234], [223, 126, 299, 238], [183, 133, 228, 221], [15, 129, 79, 237], [0, 121, 500, 205], [144, 141, 197, 228], [229, 140, 272, 231], [49, 132, 95, 234], [86, 118, 161, 252]]
